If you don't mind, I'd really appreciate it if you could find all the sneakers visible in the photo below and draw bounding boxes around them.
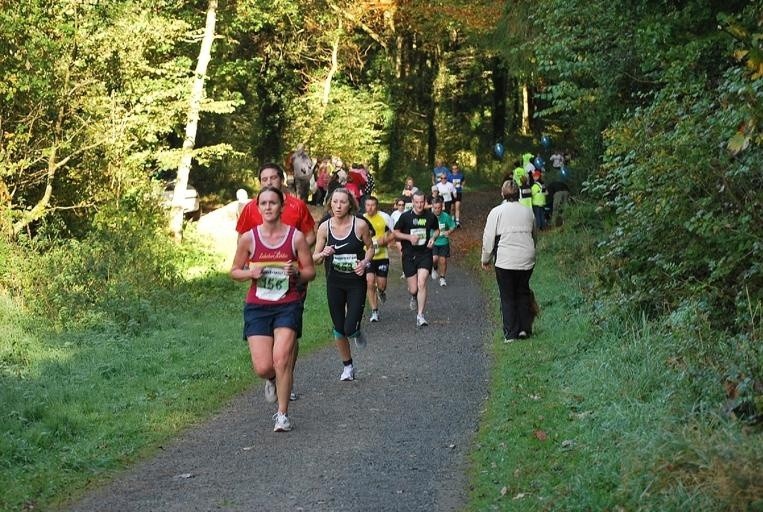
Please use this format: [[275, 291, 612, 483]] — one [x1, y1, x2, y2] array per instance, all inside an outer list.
[[370, 311, 381, 321], [377, 289, 386, 302], [416, 313, 428, 326], [265, 380, 277, 404], [355, 336, 366, 350], [289, 391, 296, 400], [273, 411, 291, 432], [456, 221, 460, 227], [516, 329, 526, 337], [503, 336, 514, 343], [340, 364, 353, 381], [431, 267, 438, 279], [439, 277, 446, 287], [410, 296, 417, 310]]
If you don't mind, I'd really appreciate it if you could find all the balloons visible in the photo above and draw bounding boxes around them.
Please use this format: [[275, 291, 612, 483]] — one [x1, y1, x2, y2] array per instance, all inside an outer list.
[[495, 143, 504, 157], [541, 135, 549, 148], [535, 158, 543, 170], [560, 165, 568, 178]]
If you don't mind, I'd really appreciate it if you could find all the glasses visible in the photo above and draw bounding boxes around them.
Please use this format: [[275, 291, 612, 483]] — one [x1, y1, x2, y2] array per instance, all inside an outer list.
[[441, 177, 447, 179]]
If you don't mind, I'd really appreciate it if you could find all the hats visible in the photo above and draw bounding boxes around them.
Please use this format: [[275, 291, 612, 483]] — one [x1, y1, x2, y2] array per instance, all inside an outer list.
[[532, 171, 540, 176], [336, 161, 343, 167]]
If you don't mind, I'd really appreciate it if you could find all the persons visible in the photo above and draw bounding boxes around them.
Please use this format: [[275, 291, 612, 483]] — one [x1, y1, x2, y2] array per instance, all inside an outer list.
[[361, 196, 395, 322], [236, 188, 248, 222], [314, 157, 322, 182], [503, 171, 513, 181], [424, 186, 445, 213], [447, 163, 465, 228], [546, 181, 569, 225], [512, 161, 525, 185], [311, 187, 375, 381], [402, 176, 418, 195], [526, 158, 535, 173], [326, 175, 342, 204], [230, 187, 316, 432], [235, 163, 316, 400], [390, 198, 408, 279], [431, 159, 451, 186], [480, 180, 537, 343], [531, 170, 546, 229], [290, 143, 313, 203], [393, 190, 440, 327], [521, 148, 535, 168], [549, 148, 564, 169], [317, 191, 375, 239], [316, 155, 333, 206], [364, 171, 375, 199], [436, 174, 457, 214], [518, 176, 532, 207], [534, 151, 545, 172], [431, 199, 456, 287], [343, 172, 368, 203], [394, 186, 413, 210]]
